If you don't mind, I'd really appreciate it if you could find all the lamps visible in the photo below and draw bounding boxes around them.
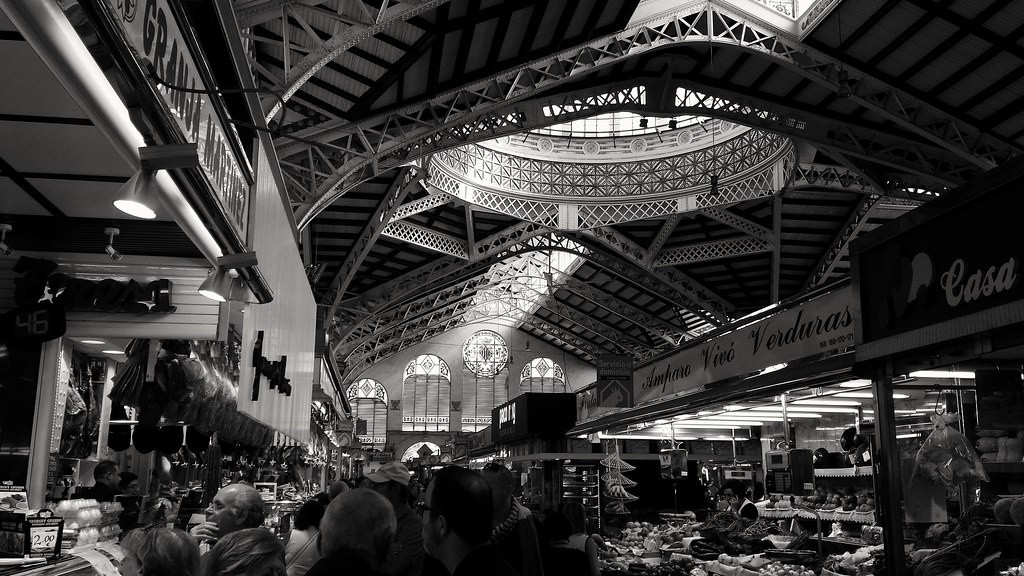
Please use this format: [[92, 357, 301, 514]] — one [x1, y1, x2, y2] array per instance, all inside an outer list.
[[197, 251, 258, 303], [510, 356, 513, 366], [526, 341, 531, 352], [112, 143, 200, 220], [0, 224, 14, 258], [710, 175, 718, 194], [104, 228, 123, 264]]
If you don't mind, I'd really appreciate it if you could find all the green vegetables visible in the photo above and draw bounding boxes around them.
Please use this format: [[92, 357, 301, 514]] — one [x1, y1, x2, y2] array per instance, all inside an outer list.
[[866, 549, 915, 576]]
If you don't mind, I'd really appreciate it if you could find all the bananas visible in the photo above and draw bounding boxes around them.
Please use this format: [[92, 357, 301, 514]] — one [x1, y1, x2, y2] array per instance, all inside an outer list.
[[606, 478, 629, 513]]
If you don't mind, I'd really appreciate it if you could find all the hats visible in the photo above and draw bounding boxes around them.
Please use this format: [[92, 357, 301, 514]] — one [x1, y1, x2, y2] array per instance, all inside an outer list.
[[363, 462, 411, 486]]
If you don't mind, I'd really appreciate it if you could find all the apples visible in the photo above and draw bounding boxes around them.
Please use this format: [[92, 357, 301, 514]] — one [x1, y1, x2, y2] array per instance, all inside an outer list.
[[616, 536, 644, 550], [795, 487, 875, 512]]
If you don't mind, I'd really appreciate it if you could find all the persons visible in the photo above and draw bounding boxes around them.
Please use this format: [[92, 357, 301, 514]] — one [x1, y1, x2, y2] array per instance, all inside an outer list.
[[118, 477, 286, 576], [285, 462, 601, 576], [720, 479, 760, 523], [85, 461, 139, 509]]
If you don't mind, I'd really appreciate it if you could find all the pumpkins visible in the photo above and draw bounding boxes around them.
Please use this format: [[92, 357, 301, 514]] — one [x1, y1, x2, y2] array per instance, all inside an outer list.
[[993, 496, 1024, 526]]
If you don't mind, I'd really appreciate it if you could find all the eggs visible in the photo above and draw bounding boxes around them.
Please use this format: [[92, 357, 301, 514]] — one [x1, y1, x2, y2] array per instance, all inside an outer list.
[[58, 498, 101, 545]]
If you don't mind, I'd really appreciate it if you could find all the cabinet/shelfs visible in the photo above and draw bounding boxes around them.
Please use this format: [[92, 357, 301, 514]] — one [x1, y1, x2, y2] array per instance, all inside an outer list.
[[516, 457, 600, 530]]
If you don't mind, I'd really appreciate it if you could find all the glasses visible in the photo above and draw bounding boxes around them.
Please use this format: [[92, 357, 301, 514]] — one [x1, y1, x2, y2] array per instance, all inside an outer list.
[[143, 518, 179, 535], [415, 504, 445, 517], [485, 462, 511, 485]]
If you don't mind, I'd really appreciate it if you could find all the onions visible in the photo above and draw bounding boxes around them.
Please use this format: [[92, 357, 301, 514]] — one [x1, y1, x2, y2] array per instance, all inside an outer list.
[[758, 560, 814, 576]]
